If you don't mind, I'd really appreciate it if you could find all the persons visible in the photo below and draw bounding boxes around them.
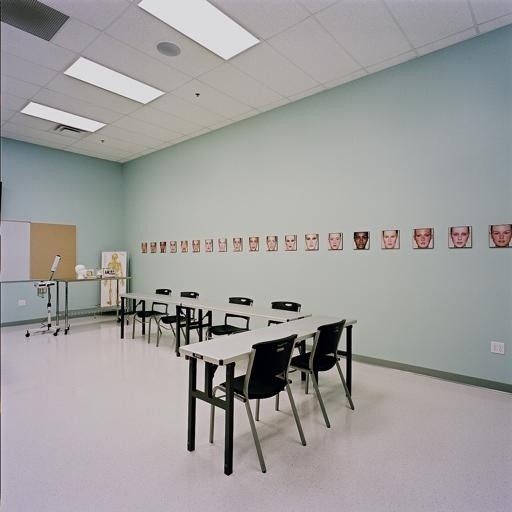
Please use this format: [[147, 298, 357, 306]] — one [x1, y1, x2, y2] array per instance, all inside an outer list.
[[382, 230, 398, 249], [450, 226, 470, 248], [142, 242, 157, 253], [193, 239, 227, 252], [491, 225, 511, 248], [305, 234, 318, 250], [353, 232, 368, 250], [285, 235, 296, 251], [160, 241, 188, 253], [328, 233, 341, 250], [233, 236, 277, 251], [413, 228, 432, 248]]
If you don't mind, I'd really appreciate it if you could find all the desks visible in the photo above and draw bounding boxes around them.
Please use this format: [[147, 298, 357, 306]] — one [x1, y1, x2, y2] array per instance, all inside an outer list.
[[116, 291, 313, 382], [48, 276, 133, 336], [178, 313, 359, 474]]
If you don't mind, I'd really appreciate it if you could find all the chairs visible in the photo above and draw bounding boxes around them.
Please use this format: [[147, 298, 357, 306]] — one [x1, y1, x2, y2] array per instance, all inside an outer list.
[[266, 301, 304, 329], [205, 296, 253, 342], [292, 317, 356, 429], [208, 333, 307, 473], [134, 289, 175, 342], [155, 290, 201, 346]]
